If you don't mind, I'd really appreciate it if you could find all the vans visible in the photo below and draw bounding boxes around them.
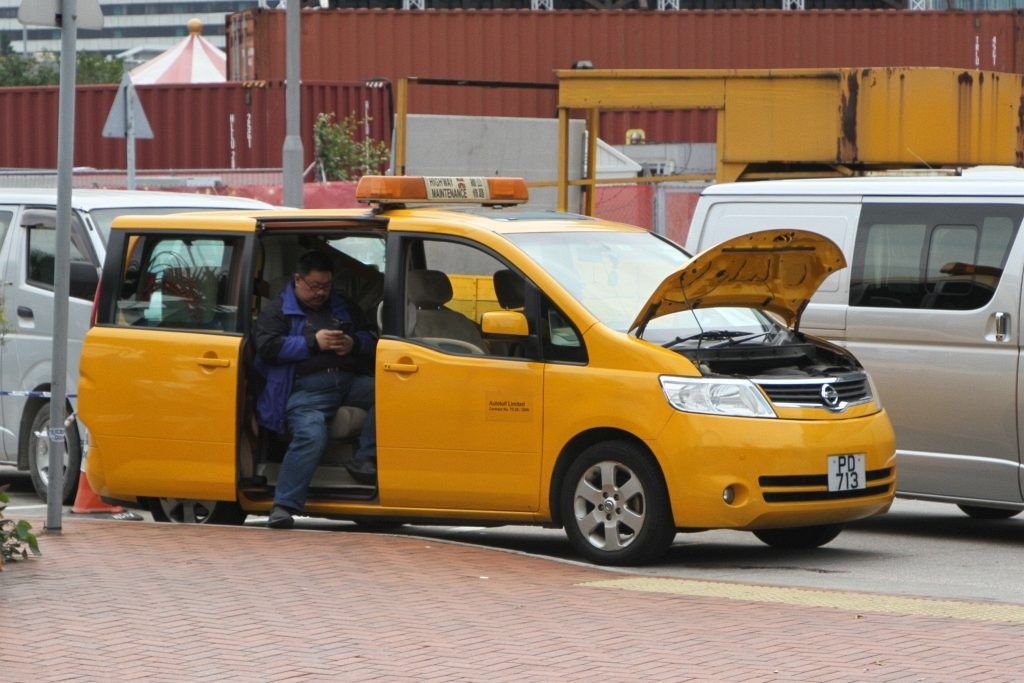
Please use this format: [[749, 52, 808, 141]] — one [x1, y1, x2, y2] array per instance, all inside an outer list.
[[0, 186, 299, 508], [77, 174, 898, 568], [686, 175, 1024, 513]]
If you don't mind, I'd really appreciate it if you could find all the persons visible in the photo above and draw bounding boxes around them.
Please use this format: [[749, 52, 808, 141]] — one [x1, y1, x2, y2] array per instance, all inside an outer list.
[[254, 249, 376, 528]]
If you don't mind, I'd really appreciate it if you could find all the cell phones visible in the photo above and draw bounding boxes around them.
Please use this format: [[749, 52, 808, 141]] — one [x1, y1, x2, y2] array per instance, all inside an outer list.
[[336, 320, 353, 335]]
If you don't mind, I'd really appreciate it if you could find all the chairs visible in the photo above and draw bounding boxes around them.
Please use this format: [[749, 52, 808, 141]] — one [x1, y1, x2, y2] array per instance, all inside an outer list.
[[260, 269, 484, 438]]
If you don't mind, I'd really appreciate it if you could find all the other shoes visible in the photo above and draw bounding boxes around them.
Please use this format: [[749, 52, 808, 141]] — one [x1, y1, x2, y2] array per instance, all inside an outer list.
[[347, 458, 377, 474], [268, 509, 293, 526]]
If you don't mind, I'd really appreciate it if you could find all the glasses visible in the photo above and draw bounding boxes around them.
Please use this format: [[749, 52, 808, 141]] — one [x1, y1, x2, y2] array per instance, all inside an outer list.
[[301, 275, 334, 292]]
